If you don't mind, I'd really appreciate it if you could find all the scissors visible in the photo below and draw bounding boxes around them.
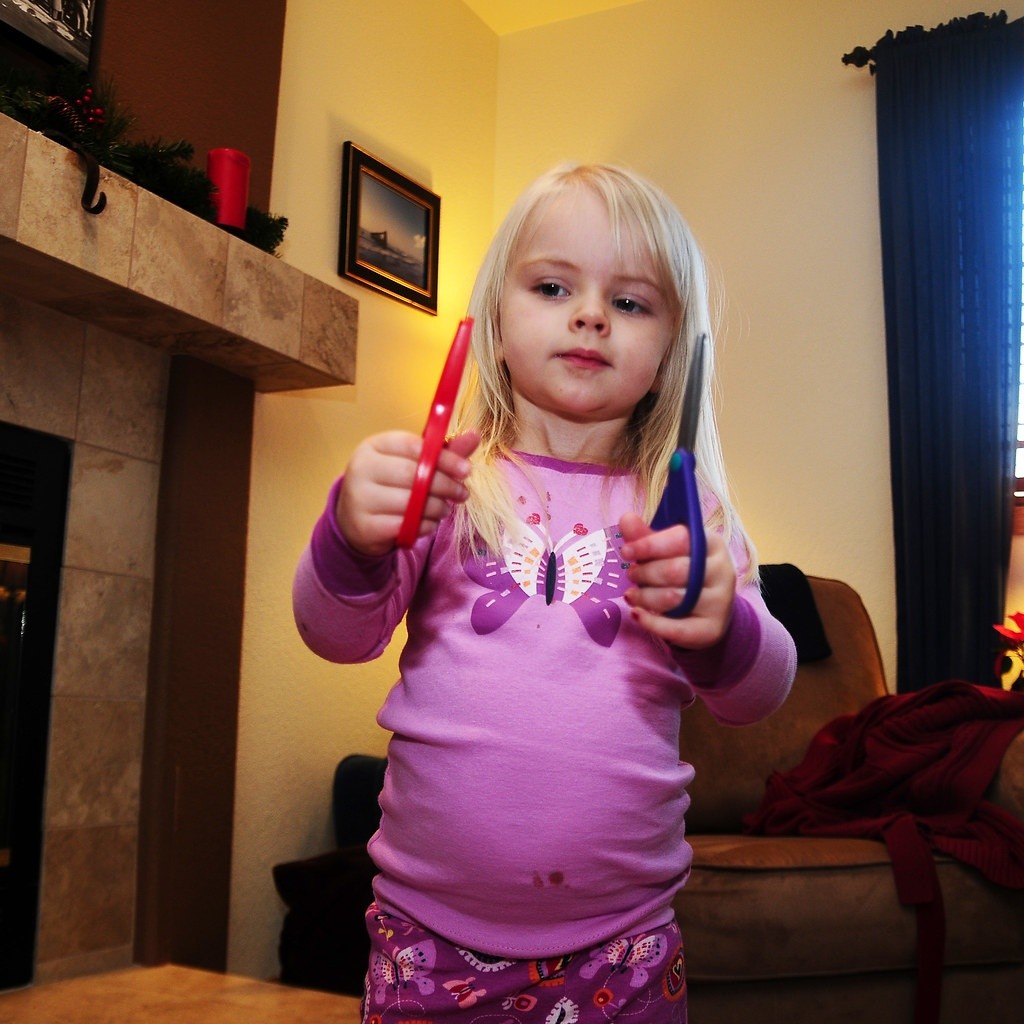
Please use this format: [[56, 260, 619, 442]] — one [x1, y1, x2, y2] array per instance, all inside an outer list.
[[393, 314, 475, 548], [635, 333, 708, 620]]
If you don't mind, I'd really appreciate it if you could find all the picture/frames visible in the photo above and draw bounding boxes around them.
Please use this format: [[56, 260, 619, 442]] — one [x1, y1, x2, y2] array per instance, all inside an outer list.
[[339, 140, 440, 323]]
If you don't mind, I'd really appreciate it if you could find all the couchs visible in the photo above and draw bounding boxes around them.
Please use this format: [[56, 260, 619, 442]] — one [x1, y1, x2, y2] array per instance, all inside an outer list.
[[668, 568, 1024, 1024]]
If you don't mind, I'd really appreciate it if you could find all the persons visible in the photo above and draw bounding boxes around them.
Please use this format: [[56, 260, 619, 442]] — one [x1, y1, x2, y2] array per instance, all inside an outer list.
[[292, 163, 798, 1024]]
